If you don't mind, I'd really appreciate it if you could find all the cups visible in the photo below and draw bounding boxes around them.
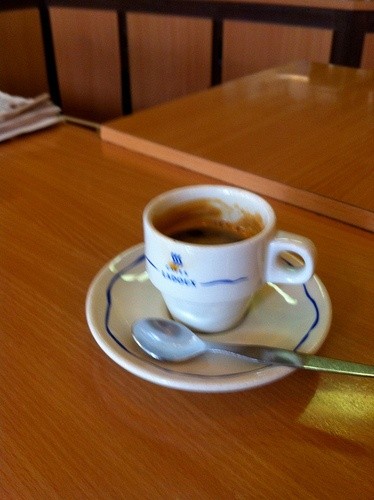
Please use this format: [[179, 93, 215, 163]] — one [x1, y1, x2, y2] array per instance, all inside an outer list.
[[143, 181, 317, 332]]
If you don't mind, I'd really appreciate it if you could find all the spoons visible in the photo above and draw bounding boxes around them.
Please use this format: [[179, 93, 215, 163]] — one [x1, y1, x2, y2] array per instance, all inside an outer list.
[[130, 317, 374, 384]]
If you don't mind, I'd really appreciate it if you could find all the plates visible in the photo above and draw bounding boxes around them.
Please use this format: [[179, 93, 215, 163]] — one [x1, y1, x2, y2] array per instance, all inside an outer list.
[[84, 237, 333, 392]]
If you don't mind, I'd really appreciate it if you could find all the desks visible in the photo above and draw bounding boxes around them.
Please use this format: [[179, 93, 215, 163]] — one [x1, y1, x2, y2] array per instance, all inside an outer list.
[[99, 58, 374, 232], [0, 122, 374, 500]]
